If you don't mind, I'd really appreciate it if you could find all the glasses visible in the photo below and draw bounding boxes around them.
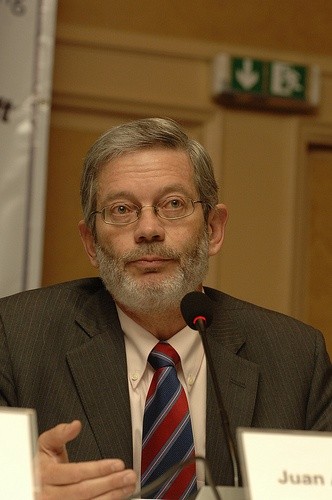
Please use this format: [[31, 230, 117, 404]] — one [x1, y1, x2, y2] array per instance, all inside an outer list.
[[89, 196, 214, 226]]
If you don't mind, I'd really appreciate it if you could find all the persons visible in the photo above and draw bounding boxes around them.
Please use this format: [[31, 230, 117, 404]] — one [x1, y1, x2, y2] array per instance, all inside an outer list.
[[1, 118, 332, 500]]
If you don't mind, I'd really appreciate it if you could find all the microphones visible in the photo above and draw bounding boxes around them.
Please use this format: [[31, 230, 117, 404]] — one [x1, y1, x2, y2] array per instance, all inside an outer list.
[[179, 290, 238, 489]]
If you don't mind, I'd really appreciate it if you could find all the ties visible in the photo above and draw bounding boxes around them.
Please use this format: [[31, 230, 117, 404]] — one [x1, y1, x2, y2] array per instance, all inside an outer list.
[[140, 342, 197, 500]]
